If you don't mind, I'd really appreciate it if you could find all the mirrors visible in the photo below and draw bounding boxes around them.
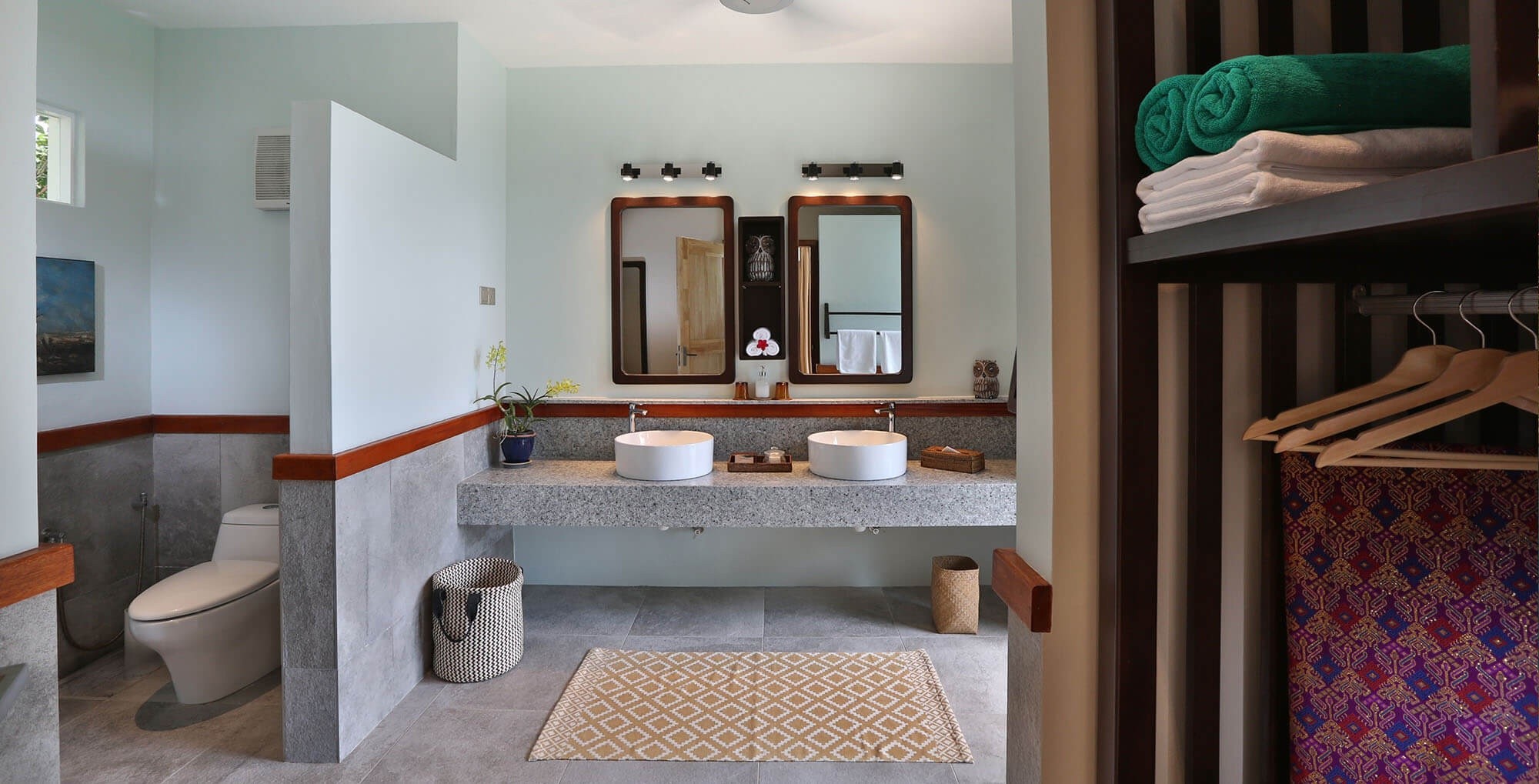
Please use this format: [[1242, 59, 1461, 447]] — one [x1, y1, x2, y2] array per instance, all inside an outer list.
[[787, 194, 914, 384], [611, 197, 735, 385]]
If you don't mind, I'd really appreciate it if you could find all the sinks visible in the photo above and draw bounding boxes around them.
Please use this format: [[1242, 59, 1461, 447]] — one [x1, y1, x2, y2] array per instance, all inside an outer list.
[[614, 429, 714, 481], [807, 429, 907, 480]]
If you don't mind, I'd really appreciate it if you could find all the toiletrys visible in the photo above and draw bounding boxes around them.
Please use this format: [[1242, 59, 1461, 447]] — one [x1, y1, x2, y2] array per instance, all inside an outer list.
[[756, 365, 771, 398]]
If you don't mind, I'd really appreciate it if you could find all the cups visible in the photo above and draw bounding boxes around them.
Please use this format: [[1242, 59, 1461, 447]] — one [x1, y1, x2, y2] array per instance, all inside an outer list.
[[775, 382, 789, 399], [734, 381, 749, 400]]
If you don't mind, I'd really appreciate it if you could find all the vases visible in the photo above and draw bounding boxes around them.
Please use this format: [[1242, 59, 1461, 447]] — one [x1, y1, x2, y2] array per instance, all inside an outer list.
[[498, 428, 536, 468]]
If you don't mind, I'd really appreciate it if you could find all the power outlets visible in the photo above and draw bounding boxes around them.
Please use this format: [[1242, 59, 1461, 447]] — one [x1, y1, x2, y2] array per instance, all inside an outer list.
[[479, 286, 497, 306]]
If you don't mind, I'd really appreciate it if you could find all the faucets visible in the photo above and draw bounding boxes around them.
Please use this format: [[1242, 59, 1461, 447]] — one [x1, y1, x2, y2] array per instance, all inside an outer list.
[[874, 400, 896, 432], [628, 402, 649, 433]]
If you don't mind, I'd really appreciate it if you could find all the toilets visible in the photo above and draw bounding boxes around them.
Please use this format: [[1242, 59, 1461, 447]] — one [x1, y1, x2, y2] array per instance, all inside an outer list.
[[129, 501, 283, 703]]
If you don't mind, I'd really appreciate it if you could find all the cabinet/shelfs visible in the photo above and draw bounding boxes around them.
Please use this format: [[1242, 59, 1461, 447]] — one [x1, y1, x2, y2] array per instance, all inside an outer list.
[[739, 216, 787, 361], [1097, 0, 1538, 784]]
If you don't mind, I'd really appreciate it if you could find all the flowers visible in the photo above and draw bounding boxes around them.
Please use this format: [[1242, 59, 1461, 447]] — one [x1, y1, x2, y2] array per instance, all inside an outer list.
[[472, 339, 584, 445]]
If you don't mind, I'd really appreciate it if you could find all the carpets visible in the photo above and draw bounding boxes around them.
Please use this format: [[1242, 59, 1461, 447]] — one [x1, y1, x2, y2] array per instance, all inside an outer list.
[[525, 645, 976, 765]]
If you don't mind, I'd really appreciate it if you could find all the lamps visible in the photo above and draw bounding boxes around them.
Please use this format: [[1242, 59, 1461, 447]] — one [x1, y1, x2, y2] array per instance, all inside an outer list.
[[622, 161, 641, 181], [701, 163, 722, 180], [843, 161, 863, 180], [884, 162, 902, 179], [802, 161, 822, 180], [661, 163, 682, 181]]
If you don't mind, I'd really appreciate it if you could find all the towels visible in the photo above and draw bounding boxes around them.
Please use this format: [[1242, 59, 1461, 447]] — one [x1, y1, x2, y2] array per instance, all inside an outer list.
[[1137, 124, 1475, 207], [1186, 40, 1474, 156], [879, 331, 902, 374], [1134, 72, 1204, 171], [835, 330, 877, 374], [1139, 163, 1460, 236]]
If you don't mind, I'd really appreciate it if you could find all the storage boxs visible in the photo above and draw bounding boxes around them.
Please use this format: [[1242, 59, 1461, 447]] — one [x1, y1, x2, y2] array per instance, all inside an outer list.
[[931, 553, 980, 636]]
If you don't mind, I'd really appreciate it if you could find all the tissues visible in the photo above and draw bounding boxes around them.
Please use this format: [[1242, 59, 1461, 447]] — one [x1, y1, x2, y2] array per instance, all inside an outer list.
[[920, 445, 986, 474]]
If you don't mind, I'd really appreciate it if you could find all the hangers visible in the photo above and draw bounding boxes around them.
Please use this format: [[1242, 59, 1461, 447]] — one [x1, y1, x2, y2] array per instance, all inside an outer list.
[[1240, 281, 1539, 474]]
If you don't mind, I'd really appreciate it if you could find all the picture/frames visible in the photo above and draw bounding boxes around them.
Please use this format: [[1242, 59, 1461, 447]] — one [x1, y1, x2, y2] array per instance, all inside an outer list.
[[36, 252, 106, 385]]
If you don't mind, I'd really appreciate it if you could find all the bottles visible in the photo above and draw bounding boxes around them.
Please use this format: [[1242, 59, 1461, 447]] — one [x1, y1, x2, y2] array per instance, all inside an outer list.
[[763, 446, 787, 463], [755, 365, 770, 398]]
